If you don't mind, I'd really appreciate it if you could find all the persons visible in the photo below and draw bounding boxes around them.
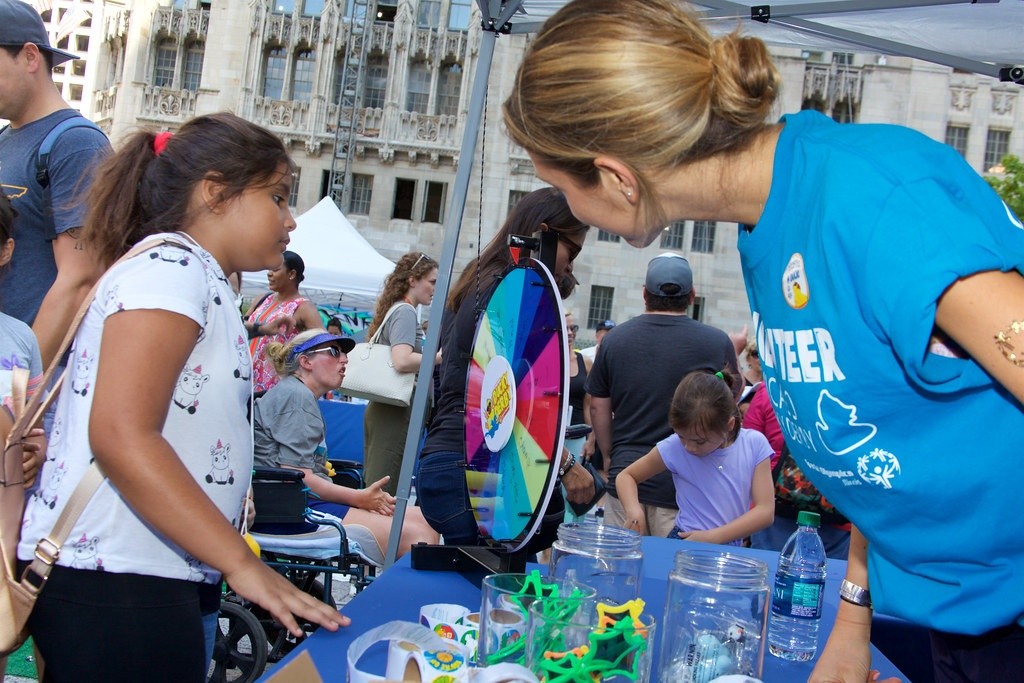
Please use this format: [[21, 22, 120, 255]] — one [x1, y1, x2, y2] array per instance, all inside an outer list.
[[0, 0, 114, 436], [0, 188, 49, 488], [16, 114, 351, 683], [229, 185, 790, 591]]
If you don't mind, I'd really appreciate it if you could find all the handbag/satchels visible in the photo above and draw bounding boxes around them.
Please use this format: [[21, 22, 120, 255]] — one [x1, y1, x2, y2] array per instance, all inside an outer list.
[[341, 302, 419, 408]]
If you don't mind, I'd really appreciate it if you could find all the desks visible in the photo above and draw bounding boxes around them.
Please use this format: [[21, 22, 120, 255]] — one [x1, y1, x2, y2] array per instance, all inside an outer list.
[[318, 399, 367, 488], [255, 538, 909, 683]]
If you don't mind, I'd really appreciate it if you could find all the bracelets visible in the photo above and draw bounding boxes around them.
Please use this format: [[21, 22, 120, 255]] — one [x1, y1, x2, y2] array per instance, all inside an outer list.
[[559, 452, 575, 477], [252, 322, 262, 335], [836, 578, 873, 608]]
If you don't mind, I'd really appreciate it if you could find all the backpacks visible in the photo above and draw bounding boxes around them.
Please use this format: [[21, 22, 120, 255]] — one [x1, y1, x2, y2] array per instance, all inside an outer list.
[[769, 442, 850, 525], [0, 238, 210, 656]]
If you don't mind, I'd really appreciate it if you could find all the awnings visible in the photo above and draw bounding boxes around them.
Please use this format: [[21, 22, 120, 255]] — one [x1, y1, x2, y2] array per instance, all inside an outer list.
[[380, 1, 1023, 577]]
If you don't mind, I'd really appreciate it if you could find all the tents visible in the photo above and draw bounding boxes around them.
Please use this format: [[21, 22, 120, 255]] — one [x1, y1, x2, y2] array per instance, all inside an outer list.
[[501, 1, 1024, 682], [235, 194, 425, 335]]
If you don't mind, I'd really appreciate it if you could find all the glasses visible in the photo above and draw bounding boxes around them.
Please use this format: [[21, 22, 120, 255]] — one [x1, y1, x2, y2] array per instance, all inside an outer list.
[[306, 344, 340, 358], [750, 350, 759, 357], [566, 325, 579, 333], [411, 252, 429, 270], [560, 236, 582, 262]]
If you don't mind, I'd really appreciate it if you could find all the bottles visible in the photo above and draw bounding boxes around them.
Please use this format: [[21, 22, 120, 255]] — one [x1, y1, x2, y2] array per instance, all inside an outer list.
[[547, 523, 643, 611], [524, 598, 657, 683], [659, 549, 771, 683], [474, 572, 597, 676], [768, 511, 828, 661]]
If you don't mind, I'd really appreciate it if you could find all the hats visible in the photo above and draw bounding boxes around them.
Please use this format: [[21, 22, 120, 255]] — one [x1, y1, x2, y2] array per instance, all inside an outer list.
[[595, 320, 617, 331], [645, 254, 693, 296], [0, 0, 81, 68], [287, 333, 356, 362]]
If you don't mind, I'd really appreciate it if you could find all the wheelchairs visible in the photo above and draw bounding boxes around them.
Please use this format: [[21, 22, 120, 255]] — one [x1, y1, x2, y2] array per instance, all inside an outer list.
[[207, 459, 377, 683]]
